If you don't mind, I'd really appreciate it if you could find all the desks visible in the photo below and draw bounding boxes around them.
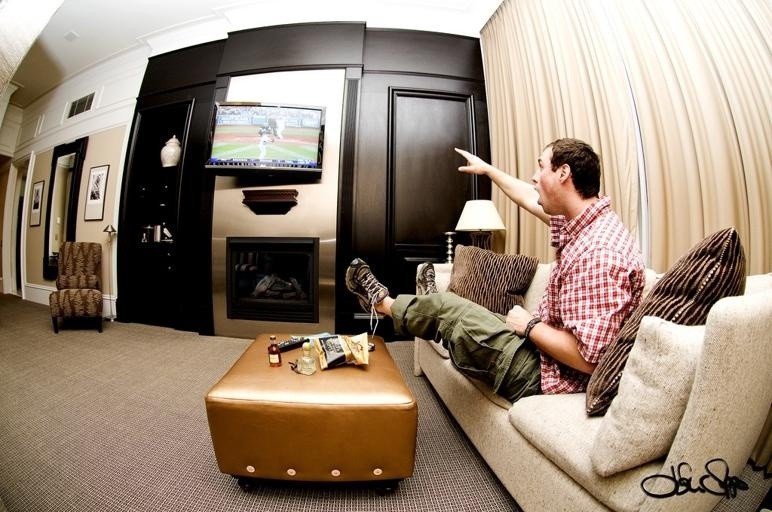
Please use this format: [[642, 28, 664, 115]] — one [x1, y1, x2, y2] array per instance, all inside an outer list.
[[203, 333, 420, 492]]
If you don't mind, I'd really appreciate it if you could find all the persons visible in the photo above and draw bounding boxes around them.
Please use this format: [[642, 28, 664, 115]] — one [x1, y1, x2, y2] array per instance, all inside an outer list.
[[344, 137, 647, 402], [258, 115, 287, 160]]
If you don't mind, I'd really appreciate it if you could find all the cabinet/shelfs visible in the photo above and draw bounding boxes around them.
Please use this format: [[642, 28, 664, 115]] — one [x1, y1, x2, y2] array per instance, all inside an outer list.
[[116, 23, 492, 328]]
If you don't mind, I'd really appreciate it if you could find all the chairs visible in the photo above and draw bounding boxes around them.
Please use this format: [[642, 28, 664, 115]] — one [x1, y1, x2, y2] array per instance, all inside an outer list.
[[50, 242, 105, 334]]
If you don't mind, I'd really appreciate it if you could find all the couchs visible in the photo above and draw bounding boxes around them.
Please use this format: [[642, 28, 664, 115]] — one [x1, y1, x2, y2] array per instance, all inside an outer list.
[[414, 264, 771, 507]]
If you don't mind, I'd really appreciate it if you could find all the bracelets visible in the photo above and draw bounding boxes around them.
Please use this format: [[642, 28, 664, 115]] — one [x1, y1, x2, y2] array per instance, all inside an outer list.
[[525, 317, 542, 338]]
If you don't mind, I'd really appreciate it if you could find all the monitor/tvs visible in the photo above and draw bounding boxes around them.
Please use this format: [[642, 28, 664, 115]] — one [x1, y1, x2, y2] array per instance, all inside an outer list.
[[205, 102, 326, 172]]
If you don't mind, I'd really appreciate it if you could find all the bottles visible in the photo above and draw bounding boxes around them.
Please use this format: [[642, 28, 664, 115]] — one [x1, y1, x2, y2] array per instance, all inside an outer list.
[[298, 343, 315, 376], [268, 336, 281, 368]]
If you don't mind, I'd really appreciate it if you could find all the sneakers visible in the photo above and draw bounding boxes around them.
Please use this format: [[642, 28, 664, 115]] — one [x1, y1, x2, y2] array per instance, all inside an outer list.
[[345, 258, 389, 315], [416, 261, 438, 295]]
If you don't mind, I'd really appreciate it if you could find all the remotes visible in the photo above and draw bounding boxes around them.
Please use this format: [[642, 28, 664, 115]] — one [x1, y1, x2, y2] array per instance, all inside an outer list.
[[278, 337, 309, 351]]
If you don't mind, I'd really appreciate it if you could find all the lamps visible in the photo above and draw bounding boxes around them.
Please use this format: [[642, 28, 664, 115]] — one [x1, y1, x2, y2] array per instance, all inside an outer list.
[[454, 199, 504, 248], [103, 226, 117, 321]]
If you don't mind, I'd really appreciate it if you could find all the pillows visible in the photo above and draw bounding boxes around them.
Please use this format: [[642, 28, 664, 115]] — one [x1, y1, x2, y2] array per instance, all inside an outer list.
[[449, 244, 538, 316], [587, 227, 745, 415], [592, 315, 717, 478]]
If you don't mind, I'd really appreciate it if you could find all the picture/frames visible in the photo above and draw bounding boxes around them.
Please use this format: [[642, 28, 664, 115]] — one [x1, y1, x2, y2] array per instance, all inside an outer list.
[[84, 165, 108, 220], [30, 182, 43, 226]]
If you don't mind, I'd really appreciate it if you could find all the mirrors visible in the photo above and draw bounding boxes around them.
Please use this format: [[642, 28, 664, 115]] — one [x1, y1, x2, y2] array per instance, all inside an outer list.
[[44, 136, 87, 279]]
